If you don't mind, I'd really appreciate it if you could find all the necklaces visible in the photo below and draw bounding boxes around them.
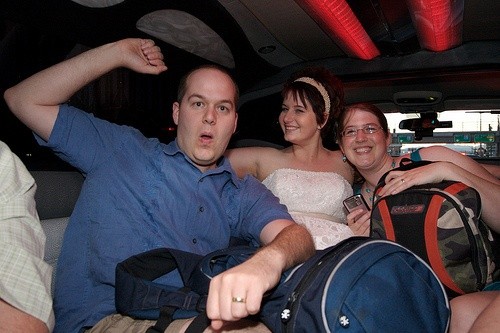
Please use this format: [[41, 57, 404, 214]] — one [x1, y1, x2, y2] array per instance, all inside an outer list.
[[361, 158, 396, 207]]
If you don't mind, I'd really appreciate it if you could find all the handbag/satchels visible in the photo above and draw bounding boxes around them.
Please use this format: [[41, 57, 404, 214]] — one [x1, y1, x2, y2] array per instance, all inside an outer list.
[[114, 236, 451, 333]]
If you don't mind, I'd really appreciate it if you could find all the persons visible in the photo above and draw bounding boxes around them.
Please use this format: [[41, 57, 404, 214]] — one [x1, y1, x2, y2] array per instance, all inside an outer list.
[[0, 139, 56, 333], [409, 116, 440, 128], [337, 102, 500, 333], [3, 36, 315, 333], [222, 68, 500, 251]]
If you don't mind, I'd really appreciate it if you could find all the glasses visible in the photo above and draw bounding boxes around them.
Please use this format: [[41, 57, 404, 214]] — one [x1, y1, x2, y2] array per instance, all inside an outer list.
[[339, 125, 384, 136]]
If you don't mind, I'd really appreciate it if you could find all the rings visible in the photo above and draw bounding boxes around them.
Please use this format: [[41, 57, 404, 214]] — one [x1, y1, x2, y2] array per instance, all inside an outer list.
[[232, 296, 246, 304], [401, 179, 405, 184]]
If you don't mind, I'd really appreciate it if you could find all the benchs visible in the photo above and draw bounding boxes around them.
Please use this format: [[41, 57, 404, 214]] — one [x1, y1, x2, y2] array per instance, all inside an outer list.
[[20, 172, 88, 301]]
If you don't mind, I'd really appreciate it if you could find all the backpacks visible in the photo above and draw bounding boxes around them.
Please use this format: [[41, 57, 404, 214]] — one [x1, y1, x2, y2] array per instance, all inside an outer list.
[[368, 157, 498, 298]]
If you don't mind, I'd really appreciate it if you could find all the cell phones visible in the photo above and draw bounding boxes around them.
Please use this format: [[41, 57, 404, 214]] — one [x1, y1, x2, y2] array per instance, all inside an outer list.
[[343, 193, 371, 222]]
